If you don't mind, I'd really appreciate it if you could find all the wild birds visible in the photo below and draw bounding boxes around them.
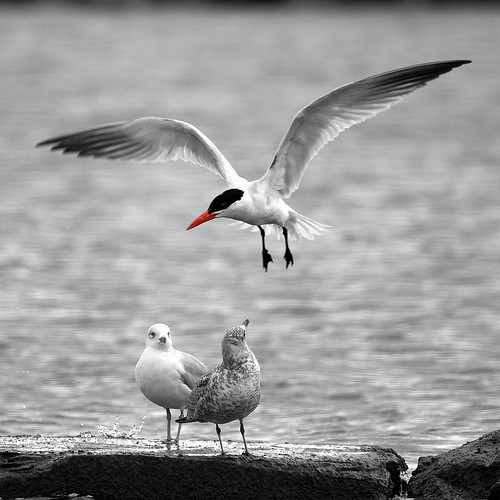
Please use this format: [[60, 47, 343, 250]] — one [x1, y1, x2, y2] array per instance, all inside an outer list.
[[134, 323, 212, 444], [33, 60, 472, 273], [174, 319, 261, 456]]
